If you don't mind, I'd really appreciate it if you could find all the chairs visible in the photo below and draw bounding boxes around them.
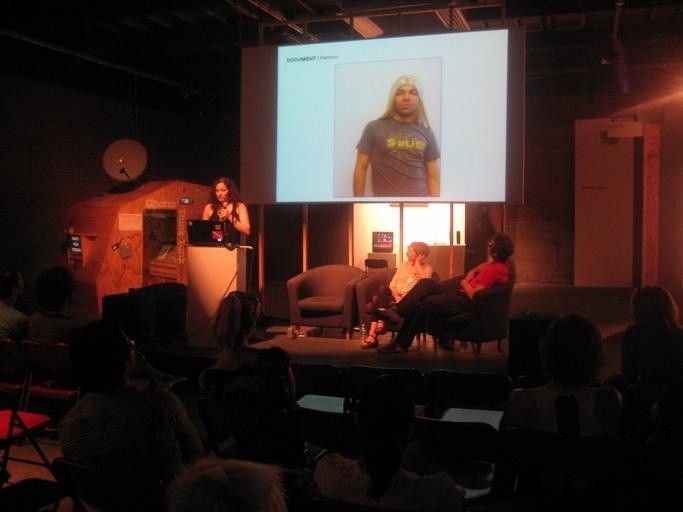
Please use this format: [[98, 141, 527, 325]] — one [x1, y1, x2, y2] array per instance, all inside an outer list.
[[355, 267, 440, 351], [438, 259, 517, 357], [287, 264, 366, 341]]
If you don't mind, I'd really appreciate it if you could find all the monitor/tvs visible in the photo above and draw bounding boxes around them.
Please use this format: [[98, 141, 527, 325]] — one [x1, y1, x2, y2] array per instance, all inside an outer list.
[[373, 232, 393, 253], [186, 219, 225, 245]]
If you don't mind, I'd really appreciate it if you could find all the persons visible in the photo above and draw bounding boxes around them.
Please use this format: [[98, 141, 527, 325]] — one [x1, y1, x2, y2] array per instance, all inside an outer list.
[[199, 177, 251, 243], [368, 233, 513, 354], [350, 75, 439, 198], [360, 242, 434, 349], [0, 266, 682, 512]]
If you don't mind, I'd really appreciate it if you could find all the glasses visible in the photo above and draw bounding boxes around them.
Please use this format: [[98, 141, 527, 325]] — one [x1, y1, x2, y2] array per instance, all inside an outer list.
[[487, 239, 493, 249]]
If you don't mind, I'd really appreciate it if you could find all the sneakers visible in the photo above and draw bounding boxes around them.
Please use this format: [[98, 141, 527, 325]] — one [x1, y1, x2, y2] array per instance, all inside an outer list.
[[376, 308, 404, 330], [377, 341, 409, 353]]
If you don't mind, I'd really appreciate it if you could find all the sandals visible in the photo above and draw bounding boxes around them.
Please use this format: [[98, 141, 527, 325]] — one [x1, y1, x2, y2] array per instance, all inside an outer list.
[[361, 334, 379, 349], [375, 321, 386, 335]]
